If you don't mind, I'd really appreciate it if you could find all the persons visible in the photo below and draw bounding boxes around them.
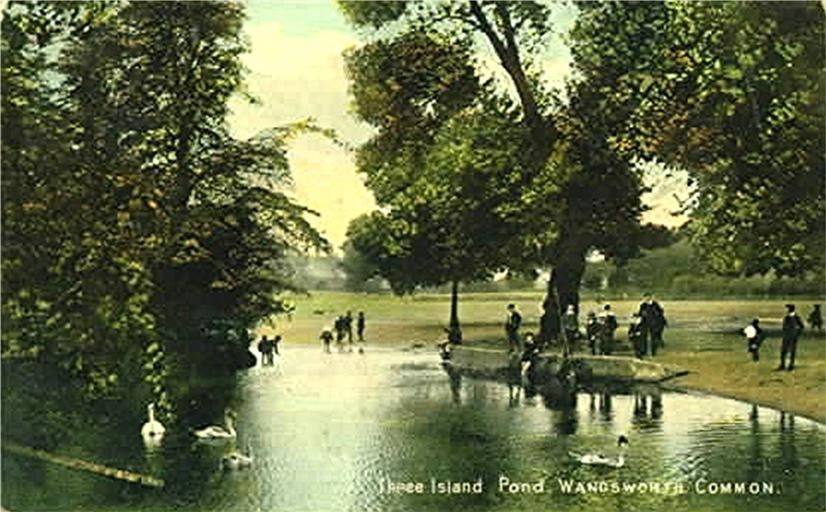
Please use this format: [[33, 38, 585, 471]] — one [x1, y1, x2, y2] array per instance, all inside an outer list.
[[335, 316, 345, 344], [506, 380, 663, 438], [343, 310, 354, 344], [807, 302, 824, 335], [774, 302, 805, 371], [739, 318, 765, 362], [443, 364, 463, 404], [318, 330, 334, 355], [503, 292, 670, 377], [356, 311, 366, 342], [443, 320, 462, 359], [258, 334, 271, 366], [266, 334, 281, 366]]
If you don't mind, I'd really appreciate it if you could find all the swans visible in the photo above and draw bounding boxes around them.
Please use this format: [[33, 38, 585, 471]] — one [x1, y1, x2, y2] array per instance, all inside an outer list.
[[188, 408, 239, 439], [140, 402, 165, 438], [568, 435, 631, 468], [220, 430, 259, 471]]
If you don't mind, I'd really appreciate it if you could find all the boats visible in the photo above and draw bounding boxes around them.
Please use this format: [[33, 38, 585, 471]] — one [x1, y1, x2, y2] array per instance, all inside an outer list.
[[440, 344, 689, 384]]
